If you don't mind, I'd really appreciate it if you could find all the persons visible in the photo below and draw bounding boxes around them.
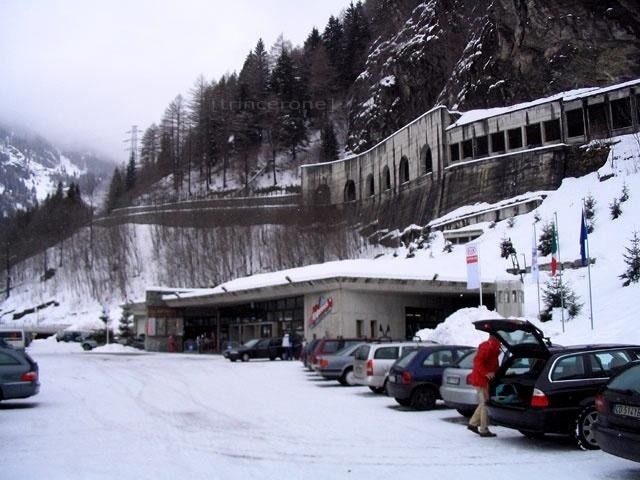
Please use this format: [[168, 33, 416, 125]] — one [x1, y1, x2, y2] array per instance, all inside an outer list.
[[467, 331, 513, 437], [282, 331, 331, 368]]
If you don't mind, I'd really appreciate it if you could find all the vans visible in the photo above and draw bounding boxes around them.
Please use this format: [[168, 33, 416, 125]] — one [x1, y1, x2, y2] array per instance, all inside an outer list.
[[0, 329, 25, 352], [223, 336, 303, 361], [355, 343, 444, 393]]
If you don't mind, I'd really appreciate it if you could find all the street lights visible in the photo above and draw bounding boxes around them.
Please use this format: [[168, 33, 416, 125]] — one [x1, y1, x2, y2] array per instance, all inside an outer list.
[[106, 298, 118, 344], [228, 135, 248, 192], [273, 114, 290, 185]]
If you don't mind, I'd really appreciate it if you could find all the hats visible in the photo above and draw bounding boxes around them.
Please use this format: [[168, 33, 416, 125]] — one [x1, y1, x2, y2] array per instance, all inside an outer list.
[[498, 330, 513, 343]]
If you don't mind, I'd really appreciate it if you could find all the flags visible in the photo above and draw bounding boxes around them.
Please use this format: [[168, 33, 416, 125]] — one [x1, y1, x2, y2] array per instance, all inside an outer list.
[[579, 207, 587, 266], [531, 227, 538, 280], [551, 220, 557, 277]]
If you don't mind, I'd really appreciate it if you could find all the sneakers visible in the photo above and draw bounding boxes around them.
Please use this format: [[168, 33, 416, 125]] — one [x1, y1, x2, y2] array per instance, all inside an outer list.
[[468, 425, 480, 434], [480, 432, 497, 437]]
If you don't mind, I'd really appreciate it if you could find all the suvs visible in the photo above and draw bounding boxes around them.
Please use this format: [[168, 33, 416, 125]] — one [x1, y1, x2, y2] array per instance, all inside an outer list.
[[472, 319, 639, 450], [0, 337, 40, 401], [57, 327, 145, 351]]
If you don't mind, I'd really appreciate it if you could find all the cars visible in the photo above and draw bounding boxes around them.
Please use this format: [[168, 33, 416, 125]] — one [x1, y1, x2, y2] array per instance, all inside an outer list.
[[592, 360, 640, 463], [304, 335, 370, 386], [24, 331, 33, 347], [440, 344, 564, 417], [388, 346, 476, 411]]
